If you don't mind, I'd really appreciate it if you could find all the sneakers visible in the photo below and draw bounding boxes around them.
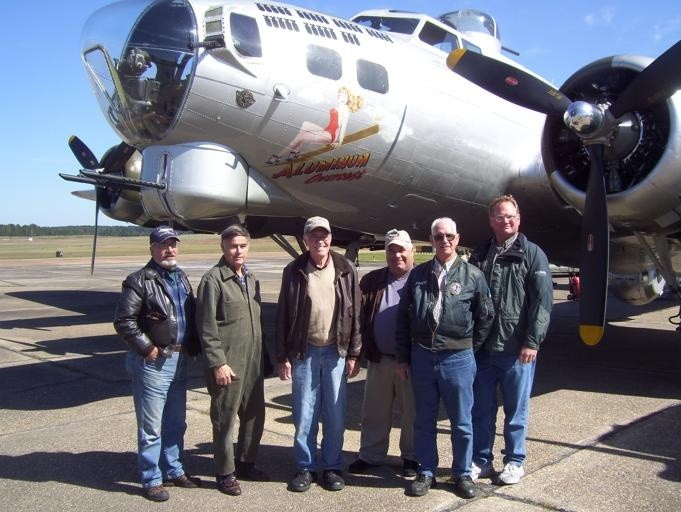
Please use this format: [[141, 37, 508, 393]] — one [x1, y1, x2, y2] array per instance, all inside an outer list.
[[347, 458, 386, 475], [493, 461, 528, 486], [470, 457, 495, 479], [163, 472, 204, 490], [402, 457, 420, 480], [140, 485, 172, 503]]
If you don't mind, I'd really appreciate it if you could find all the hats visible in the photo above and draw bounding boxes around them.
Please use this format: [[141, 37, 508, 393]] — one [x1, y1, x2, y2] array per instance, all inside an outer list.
[[383, 228, 414, 252], [302, 215, 334, 235], [149, 224, 181, 247]]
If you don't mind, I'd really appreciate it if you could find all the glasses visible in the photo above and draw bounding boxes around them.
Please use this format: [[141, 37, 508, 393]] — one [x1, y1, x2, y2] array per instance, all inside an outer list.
[[489, 213, 521, 224], [430, 233, 458, 243]]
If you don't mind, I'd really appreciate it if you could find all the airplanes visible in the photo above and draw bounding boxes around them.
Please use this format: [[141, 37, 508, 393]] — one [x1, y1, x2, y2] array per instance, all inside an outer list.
[[55, 0, 681, 351], [546, 264, 580, 277]]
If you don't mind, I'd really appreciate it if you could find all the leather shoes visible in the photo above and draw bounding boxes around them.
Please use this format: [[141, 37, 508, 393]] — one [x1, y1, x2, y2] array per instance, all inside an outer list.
[[235, 460, 271, 483], [216, 474, 245, 497], [453, 473, 479, 499], [288, 466, 320, 493], [321, 467, 348, 492], [405, 474, 439, 497]]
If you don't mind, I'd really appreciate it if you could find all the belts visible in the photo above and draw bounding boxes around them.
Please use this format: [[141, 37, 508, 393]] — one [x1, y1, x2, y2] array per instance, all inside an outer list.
[[173, 343, 189, 353]]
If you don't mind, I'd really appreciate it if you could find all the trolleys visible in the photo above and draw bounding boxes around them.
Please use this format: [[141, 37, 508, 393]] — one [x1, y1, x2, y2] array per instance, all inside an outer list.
[[565, 267, 580, 302]]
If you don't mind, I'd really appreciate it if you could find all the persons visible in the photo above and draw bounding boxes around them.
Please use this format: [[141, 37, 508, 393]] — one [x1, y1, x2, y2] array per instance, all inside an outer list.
[[350, 228, 417, 476], [275, 216, 366, 493], [114, 226, 202, 501], [466, 196, 554, 484], [397, 216, 495, 498], [197, 225, 274, 494]]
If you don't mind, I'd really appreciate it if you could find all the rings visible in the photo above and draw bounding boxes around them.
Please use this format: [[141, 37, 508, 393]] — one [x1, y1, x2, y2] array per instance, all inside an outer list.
[[220, 381, 223, 384]]
